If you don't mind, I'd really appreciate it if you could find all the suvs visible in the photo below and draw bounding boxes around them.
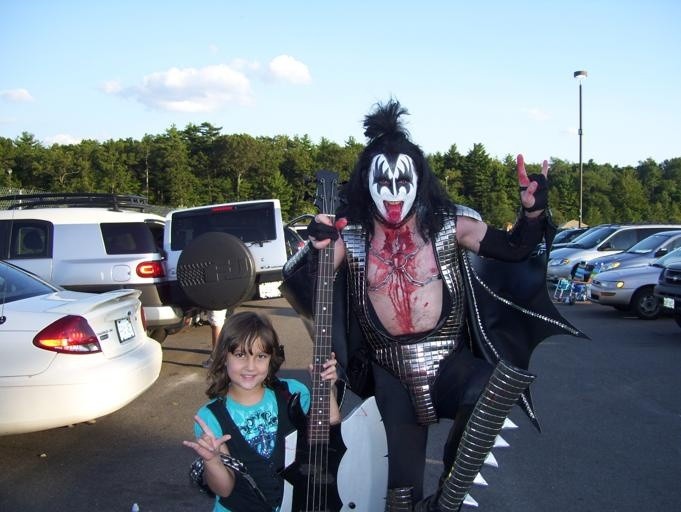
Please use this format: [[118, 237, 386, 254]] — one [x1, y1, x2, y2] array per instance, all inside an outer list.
[[655, 263, 681, 328], [0, 191, 288, 346]]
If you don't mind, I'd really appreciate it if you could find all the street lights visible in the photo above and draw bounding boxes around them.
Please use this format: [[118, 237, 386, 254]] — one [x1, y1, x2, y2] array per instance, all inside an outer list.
[[574, 67, 587, 227]]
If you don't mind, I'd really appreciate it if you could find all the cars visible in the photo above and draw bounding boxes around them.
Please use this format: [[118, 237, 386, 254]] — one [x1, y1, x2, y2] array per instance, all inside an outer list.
[[545, 224, 681, 320], [0, 259, 162, 438], [278, 223, 308, 262]]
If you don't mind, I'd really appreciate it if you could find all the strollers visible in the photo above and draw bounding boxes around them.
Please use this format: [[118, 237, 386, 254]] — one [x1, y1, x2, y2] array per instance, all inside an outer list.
[[552, 263, 589, 307]]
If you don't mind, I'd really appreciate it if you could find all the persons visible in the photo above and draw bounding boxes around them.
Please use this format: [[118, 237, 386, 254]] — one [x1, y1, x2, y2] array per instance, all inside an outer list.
[[280, 97, 592, 512], [201, 308, 227, 367], [182, 312, 341, 512]]
